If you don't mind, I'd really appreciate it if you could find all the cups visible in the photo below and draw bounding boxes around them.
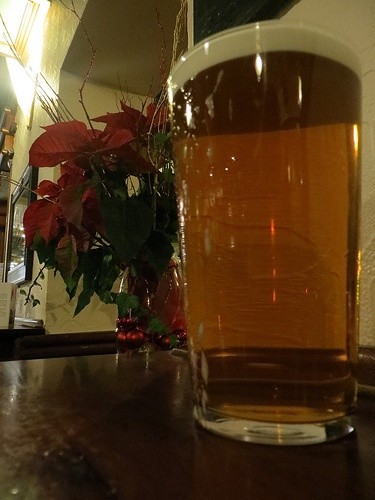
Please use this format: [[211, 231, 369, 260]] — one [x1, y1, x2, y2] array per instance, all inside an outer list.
[[166, 20, 361, 446]]
